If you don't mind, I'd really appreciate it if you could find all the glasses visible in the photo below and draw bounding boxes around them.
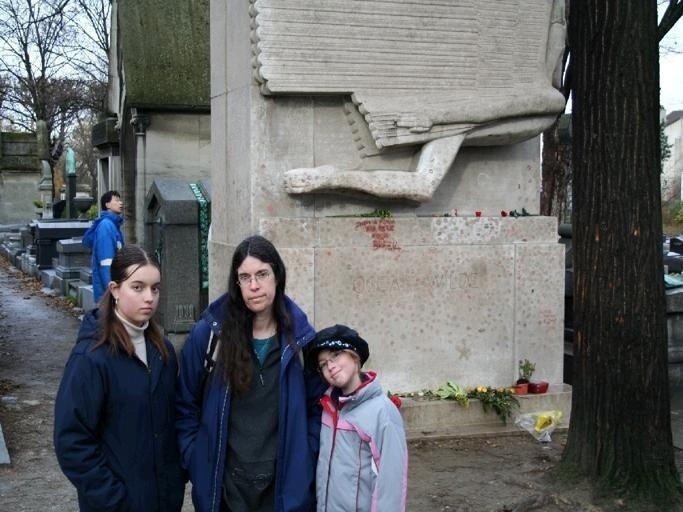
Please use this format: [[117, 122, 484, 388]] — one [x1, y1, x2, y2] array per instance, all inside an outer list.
[[236, 271, 271, 287], [316, 350, 344, 373]]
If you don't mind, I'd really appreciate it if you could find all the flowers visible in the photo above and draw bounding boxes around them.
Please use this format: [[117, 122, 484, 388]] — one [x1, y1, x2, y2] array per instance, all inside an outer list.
[[433, 380, 520, 427], [499, 208, 538, 218]]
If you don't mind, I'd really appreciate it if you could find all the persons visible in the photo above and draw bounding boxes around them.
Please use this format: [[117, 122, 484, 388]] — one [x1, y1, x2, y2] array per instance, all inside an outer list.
[[307, 325, 408, 512], [174, 234, 330, 512], [52, 243, 190, 512], [81, 191, 125, 305]]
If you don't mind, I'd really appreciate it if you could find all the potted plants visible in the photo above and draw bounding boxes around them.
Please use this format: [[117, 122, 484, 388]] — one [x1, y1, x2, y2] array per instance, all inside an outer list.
[[511, 357, 537, 395]]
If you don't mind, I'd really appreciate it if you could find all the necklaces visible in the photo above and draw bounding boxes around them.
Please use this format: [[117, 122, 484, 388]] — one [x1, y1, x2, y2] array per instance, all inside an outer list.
[[250, 326, 276, 359]]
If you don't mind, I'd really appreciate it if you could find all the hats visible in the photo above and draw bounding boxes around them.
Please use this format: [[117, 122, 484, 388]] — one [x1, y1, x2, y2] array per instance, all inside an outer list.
[[308, 324, 369, 368]]
